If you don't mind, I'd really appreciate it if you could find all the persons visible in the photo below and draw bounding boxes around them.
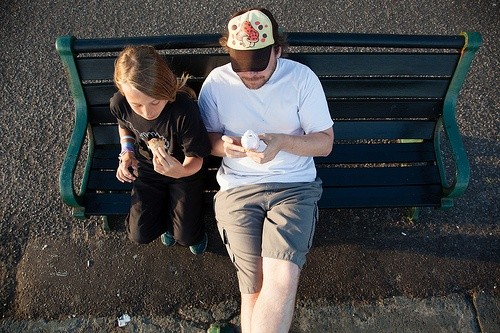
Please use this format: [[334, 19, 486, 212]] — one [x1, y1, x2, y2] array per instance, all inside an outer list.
[[109, 46, 207, 255], [197, 8, 334, 333]]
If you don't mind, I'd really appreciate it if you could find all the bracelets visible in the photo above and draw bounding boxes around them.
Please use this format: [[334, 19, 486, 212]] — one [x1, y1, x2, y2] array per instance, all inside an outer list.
[[120, 135, 135, 153]]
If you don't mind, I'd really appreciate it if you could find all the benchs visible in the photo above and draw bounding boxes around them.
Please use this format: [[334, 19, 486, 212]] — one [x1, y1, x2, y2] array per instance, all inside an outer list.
[[55, 28, 483, 234]]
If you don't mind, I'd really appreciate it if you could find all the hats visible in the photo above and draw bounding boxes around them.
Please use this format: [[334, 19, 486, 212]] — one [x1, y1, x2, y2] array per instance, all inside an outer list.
[[226, 6, 280, 72]]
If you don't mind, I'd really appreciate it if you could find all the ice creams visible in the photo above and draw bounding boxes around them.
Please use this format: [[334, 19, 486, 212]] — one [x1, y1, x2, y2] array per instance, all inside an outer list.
[[148, 138, 165, 166], [241, 130, 260, 151]]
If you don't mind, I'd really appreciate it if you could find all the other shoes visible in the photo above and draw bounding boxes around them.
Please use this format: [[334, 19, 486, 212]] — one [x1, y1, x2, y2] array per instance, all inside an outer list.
[[161, 229, 176, 247], [189, 230, 208, 255]]
